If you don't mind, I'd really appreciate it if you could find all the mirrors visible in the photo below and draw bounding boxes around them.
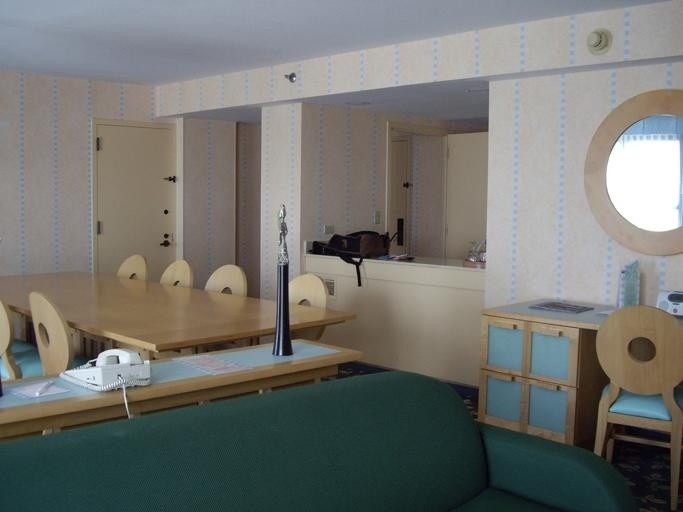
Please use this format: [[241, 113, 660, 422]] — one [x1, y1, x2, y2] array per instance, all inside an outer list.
[[584, 88, 683, 255]]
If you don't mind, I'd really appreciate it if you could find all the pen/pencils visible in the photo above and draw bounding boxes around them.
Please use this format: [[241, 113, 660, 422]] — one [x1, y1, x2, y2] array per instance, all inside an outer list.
[[36, 380, 55, 396]]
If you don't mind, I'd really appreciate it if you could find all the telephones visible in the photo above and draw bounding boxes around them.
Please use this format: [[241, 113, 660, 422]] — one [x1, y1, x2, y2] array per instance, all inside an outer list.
[[60, 348, 152, 392]]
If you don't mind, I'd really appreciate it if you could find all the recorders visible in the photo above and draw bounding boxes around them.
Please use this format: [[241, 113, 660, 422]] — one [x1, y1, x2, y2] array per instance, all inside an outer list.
[[656, 289, 683, 316]]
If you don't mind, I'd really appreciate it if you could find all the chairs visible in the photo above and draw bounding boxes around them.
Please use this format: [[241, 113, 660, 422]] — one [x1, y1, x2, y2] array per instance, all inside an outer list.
[[160, 259, 193, 289], [30, 291, 95, 375], [116, 254, 151, 281], [273, 273, 328, 342], [205, 264, 247, 296], [0, 300, 43, 381], [593, 305, 683, 512]]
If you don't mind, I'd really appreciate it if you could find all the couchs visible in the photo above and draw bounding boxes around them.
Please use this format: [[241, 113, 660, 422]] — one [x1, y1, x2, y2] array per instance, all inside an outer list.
[[0, 368, 635, 512]]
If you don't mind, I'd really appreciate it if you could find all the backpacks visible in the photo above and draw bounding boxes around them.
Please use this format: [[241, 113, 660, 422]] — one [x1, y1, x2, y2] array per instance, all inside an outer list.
[[312, 231, 390, 265]]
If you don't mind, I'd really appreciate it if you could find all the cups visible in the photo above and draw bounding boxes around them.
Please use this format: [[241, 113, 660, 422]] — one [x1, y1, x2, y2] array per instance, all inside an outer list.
[[467, 252, 486, 262]]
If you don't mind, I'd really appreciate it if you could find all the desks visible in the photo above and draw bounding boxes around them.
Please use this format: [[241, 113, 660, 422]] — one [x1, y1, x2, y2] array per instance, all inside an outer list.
[[478, 297, 683, 446], [0, 338, 362, 439]]
